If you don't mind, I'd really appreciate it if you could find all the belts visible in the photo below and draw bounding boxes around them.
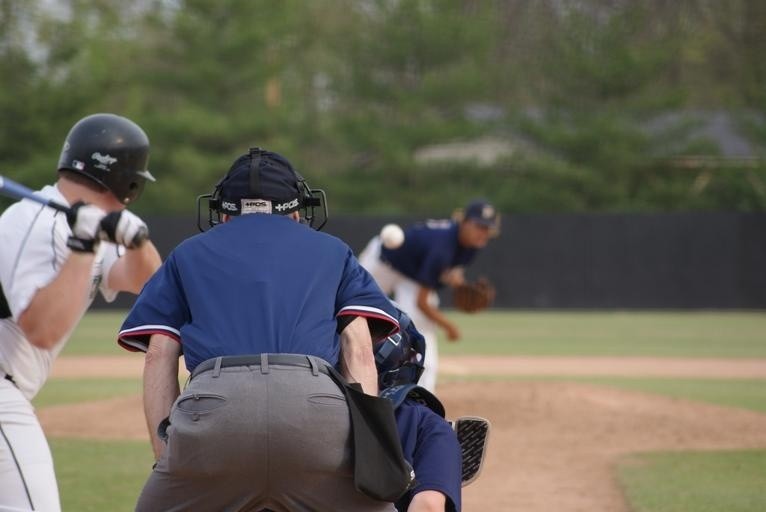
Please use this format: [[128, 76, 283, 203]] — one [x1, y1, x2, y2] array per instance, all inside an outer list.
[[189, 356, 330, 380]]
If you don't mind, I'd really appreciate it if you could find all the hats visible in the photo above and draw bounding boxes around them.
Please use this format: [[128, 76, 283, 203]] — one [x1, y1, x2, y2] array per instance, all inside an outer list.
[[465, 201, 497, 228]]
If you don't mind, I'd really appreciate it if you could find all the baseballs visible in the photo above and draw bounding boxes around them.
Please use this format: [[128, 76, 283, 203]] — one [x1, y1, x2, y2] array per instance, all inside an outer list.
[[381, 222, 404, 248]]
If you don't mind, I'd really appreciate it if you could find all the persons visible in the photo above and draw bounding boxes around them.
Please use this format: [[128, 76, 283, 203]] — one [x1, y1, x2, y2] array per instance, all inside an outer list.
[[357, 201, 501, 395], [116, 146, 404, 511], [372, 298, 464, 512], [0, 112, 164, 511]]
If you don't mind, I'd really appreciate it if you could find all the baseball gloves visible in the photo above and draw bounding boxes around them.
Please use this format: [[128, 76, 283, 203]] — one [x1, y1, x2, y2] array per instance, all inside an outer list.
[[453, 274, 495, 314]]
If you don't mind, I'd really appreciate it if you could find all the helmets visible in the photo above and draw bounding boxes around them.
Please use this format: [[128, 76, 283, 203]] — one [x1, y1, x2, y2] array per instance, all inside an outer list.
[[372, 307, 425, 389], [196, 147, 329, 233], [56, 112, 156, 205]]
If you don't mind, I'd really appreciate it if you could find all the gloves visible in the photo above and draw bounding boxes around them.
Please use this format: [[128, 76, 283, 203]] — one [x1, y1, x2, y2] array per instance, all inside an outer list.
[[100, 209, 150, 250], [64, 201, 108, 252]]
